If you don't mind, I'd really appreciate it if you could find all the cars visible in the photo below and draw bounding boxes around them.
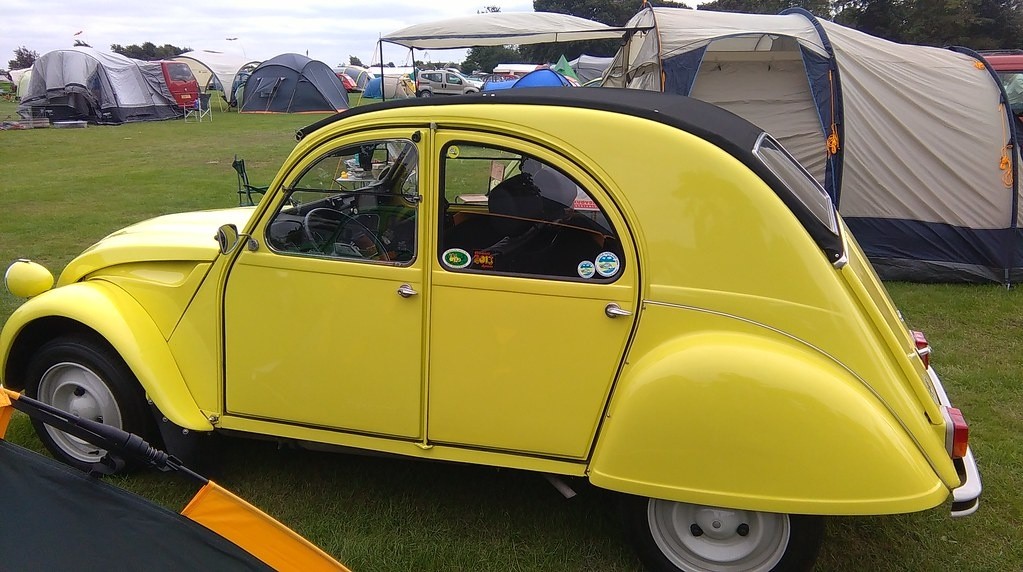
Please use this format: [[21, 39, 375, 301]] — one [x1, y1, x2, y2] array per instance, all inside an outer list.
[[0, 84, 983, 572], [416, 66, 520, 97]]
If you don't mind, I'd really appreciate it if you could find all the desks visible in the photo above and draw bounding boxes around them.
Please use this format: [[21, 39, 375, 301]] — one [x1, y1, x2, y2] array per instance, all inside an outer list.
[[336, 162, 395, 190]]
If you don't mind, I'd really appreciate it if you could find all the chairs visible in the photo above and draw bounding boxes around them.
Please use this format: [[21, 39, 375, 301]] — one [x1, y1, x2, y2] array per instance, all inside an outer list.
[[402, 170, 416, 194], [371, 198, 455, 262], [455, 160, 505, 204], [232, 154, 300, 207], [469, 169, 578, 272], [184, 93, 212, 123]]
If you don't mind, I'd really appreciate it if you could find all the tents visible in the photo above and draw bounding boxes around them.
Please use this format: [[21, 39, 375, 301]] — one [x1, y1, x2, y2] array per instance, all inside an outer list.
[[0, 384, 355, 572], [0, 0, 1023, 293]]
